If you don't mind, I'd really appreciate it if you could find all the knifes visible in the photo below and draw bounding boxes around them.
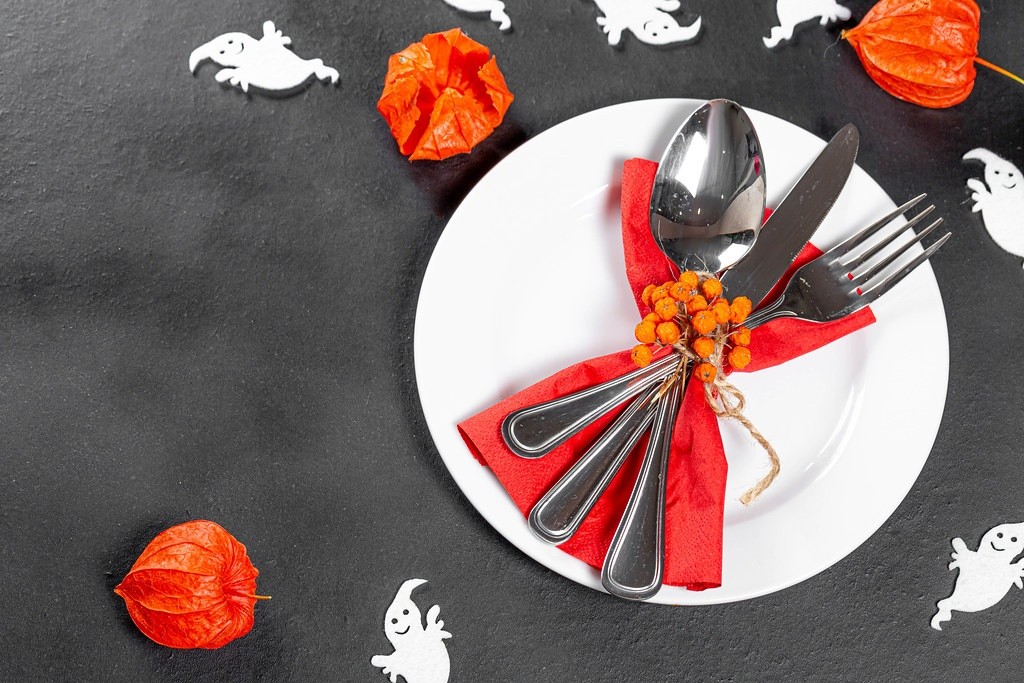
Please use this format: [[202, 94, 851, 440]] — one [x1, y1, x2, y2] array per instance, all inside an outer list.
[[527, 122, 859, 547]]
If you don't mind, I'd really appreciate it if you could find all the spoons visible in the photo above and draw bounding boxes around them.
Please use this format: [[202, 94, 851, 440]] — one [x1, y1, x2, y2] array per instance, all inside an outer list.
[[599, 97, 768, 601]]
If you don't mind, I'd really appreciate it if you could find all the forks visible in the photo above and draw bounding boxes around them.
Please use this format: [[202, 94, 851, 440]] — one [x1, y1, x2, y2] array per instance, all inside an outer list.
[[501, 192, 952, 460]]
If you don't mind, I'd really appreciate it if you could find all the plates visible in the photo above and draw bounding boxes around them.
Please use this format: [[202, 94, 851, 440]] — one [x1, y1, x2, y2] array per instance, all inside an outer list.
[[412, 99, 949, 606]]
